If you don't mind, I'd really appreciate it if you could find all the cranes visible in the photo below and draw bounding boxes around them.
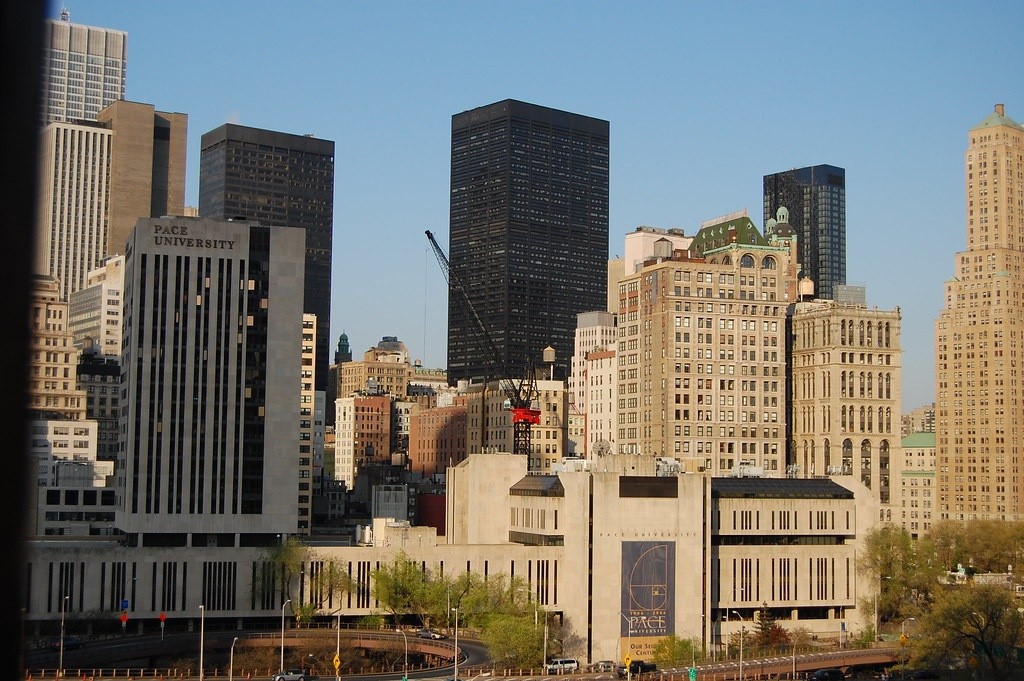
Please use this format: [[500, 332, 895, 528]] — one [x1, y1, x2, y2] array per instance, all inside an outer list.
[[422, 229, 543, 472]]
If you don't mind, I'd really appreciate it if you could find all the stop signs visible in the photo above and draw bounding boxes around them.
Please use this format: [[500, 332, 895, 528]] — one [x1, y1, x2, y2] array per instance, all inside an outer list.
[[516, 588, 537, 632]]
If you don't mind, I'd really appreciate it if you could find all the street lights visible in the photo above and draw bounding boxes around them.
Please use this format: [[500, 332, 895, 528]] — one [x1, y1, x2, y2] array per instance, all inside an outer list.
[[874, 575, 892, 642], [229, 636, 238, 681], [451, 606, 459, 681], [732, 610, 744, 681], [792, 632, 812, 679], [59, 595, 71, 670], [900, 617, 915, 681], [700, 614, 727, 662], [726, 588, 745, 661], [395, 628, 409, 681], [542, 603, 558, 668], [681, 629, 696, 668], [281, 600, 292, 672], [198, 605, 204, 681], [617, 611, 646, 681]]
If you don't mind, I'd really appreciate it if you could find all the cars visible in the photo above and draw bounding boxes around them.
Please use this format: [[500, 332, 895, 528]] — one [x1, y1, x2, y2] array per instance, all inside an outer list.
[[271, 669, 320, 681], [47, 634, 84, 652], [541, 657, 658, 678], [417, 628, 446, 640]]
